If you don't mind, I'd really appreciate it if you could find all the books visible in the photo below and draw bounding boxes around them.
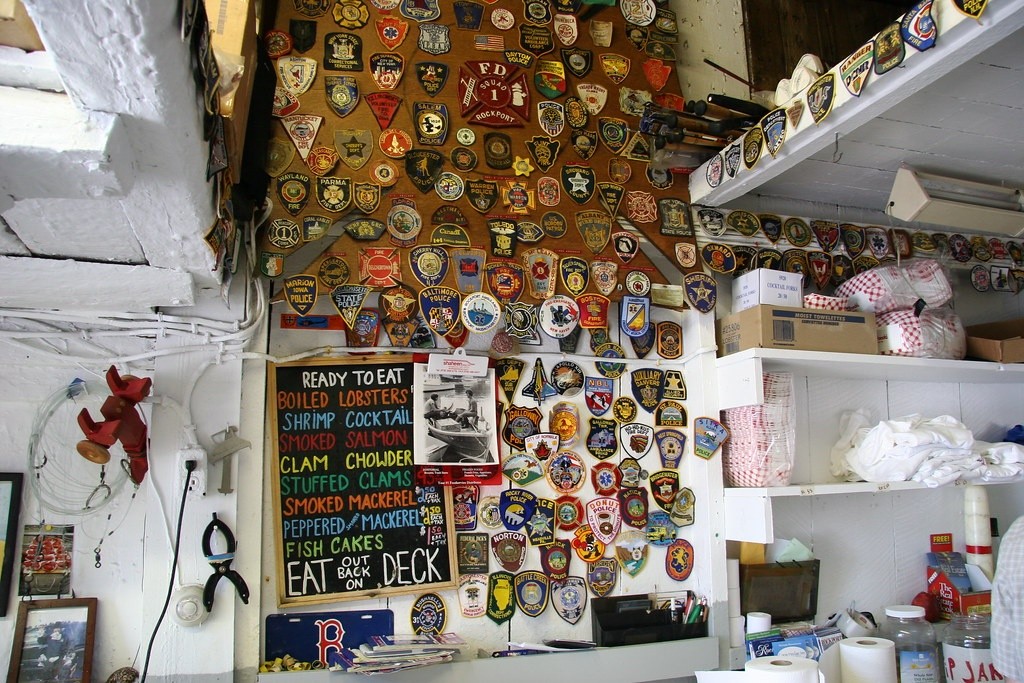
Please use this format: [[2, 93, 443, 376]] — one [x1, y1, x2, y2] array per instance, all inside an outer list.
[[331, 630, 466, 676], [481, 646, 541, 656]]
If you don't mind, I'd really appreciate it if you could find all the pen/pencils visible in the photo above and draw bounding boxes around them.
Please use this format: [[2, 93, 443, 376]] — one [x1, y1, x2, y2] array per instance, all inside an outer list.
[[671, 596, 709, 623]]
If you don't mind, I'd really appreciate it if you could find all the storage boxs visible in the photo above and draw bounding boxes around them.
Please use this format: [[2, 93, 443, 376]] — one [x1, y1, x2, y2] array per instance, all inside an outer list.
[[732, 268, 803, 313], [928, 565, 992, 622], [716, 305, 879, 359], [965, 316, 1024, 363]]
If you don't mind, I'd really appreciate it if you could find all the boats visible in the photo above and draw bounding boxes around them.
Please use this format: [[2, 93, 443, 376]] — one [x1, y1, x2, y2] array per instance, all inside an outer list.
[[424, 396, 492, 463], [421, 365, 491, 393]]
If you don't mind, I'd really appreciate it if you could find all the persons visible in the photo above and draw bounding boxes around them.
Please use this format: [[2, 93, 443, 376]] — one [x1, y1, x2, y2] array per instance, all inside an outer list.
[[990, 517, 1024, 683], [33, 622, 77, 683]]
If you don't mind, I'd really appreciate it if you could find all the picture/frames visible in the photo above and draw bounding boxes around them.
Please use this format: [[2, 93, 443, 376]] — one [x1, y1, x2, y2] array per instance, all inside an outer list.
[[6, 597, 98, 683], [0, 471, 24, 617]]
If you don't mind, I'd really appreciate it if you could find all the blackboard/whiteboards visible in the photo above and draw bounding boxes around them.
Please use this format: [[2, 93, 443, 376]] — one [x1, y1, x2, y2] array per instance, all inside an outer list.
[[268, 354, 460, 608]]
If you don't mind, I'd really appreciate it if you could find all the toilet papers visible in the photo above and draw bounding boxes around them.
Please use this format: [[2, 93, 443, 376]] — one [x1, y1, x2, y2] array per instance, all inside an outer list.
[[693, 655, 819, 683], [747, 611, 771, 634], [819, 636, 898, 683]]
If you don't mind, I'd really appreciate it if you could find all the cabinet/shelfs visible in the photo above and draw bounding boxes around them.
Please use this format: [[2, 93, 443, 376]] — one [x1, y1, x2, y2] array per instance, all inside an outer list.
[[683, 206, 1024, 682]]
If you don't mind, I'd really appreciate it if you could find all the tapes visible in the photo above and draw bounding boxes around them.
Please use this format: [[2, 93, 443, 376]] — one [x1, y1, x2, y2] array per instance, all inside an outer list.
[[836, 608, 876, 638]]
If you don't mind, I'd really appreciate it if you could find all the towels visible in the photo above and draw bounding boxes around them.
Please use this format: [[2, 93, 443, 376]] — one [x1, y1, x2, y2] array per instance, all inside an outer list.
[[829, 408, 1024, 489]]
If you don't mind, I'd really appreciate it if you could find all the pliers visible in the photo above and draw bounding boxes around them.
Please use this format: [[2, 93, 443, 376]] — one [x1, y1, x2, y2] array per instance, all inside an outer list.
[[200, 512, 251, 613]]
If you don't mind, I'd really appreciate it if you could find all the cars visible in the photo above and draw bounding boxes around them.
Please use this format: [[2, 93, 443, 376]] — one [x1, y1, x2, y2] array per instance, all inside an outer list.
[[20, 621, 86, 679]]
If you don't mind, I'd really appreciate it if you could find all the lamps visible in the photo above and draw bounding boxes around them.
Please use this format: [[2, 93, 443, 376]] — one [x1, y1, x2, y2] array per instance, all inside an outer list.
[[883, 166, 1024, 238]]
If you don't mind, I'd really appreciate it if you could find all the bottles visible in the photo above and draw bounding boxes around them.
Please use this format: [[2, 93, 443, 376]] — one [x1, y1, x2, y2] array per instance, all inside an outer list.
[[877, 605, 940, 683], [941, 613, 1005, 683]]
